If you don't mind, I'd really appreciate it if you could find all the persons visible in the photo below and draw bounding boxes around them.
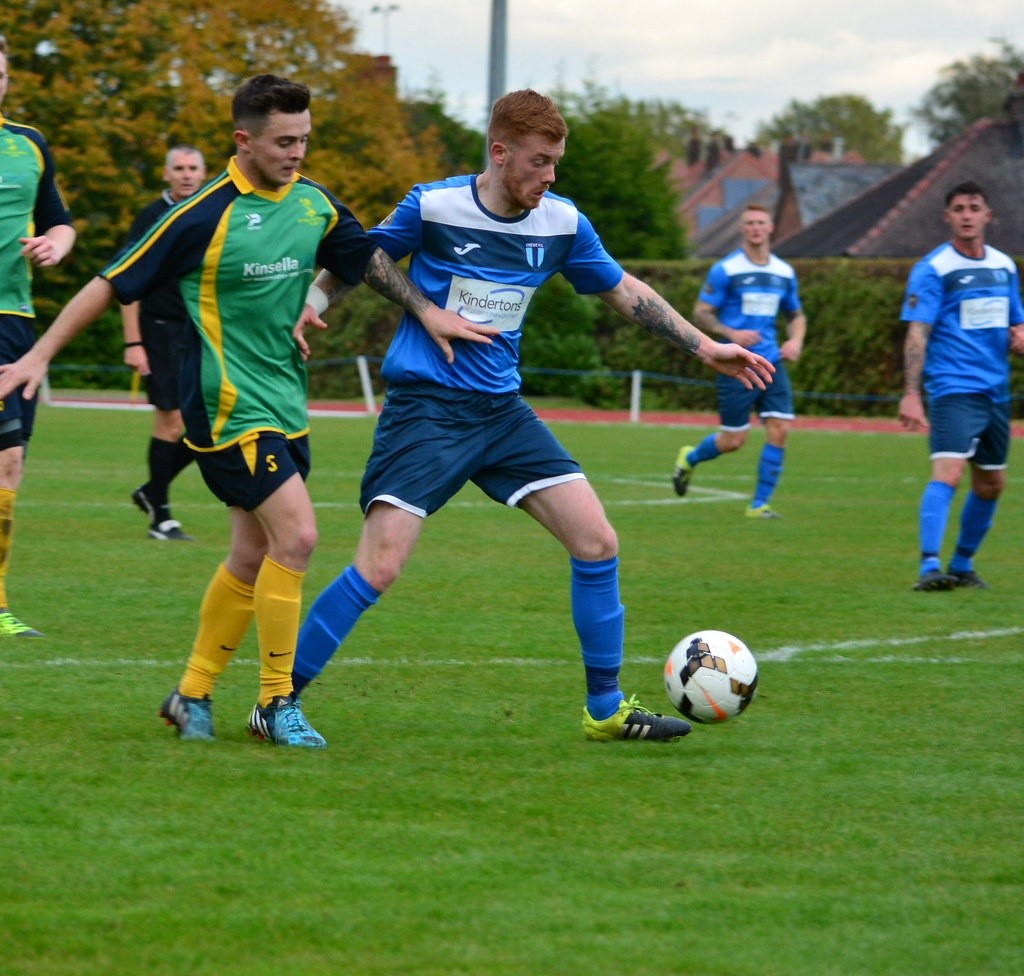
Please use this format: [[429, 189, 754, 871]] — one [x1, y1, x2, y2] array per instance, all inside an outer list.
[[0, 75, 502, 748], [292, 90, 777, 740], [1, 37, 76, 637], [118, 146, 206, 546], [672, 207, 805, 517], [899, 181, 1024, 591]]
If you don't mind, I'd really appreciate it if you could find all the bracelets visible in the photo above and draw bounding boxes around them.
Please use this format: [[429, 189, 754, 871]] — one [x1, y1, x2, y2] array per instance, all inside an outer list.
[[123, 342, 143, 348], [305, 286, 328, 314]]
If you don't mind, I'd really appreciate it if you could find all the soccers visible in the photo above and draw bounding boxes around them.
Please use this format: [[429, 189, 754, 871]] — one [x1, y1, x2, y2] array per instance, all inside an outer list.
[[664, 630, 759, 725]]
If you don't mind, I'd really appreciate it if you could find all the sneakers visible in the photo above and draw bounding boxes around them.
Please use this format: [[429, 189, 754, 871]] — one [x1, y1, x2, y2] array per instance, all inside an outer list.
[[914, 571, 959, 592], [132, 484, 155, 516], [673, 445, 695, 497], [582, 693, 692, 742], [248, 692, 328, 748], [148, 519, 193, 541], [946, 566, 988, 589], [745, 505, 781, 519], [0, 608, 46, 640], [160, 688, 215, 740]]
